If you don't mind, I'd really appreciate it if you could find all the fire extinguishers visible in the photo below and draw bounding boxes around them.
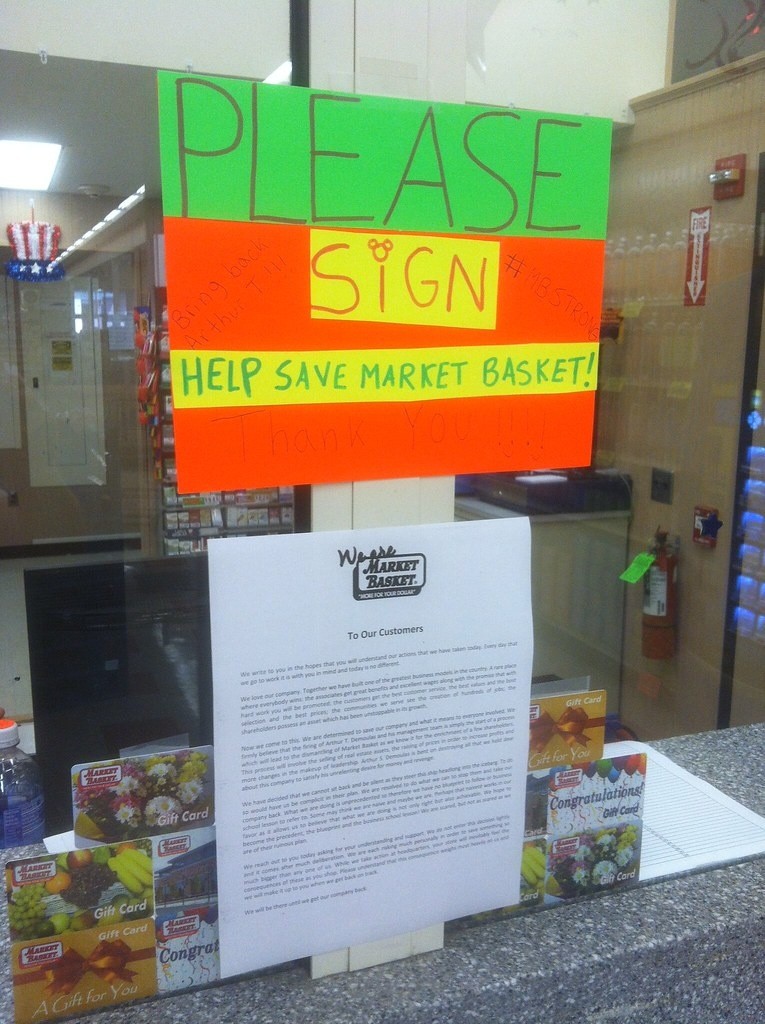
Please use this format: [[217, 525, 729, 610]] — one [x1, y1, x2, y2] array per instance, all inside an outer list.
[[641, 524, 681, 660]]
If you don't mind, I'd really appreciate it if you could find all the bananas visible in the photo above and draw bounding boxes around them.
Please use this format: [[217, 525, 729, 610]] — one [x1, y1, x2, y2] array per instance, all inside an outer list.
[[108, 847, 152, 894], [521, 846, 546, 884]]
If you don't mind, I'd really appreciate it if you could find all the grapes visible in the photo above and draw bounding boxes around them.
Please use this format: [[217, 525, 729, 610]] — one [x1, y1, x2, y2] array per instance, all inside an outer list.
[[9, 862, 118, 940]]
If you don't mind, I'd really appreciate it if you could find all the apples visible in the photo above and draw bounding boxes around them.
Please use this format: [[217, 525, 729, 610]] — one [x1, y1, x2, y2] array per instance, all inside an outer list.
[[66, 849, 93, 872]]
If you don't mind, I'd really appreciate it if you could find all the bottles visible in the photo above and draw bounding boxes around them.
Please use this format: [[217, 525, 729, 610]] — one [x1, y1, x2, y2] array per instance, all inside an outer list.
[[0, 719, 46, 851]]
